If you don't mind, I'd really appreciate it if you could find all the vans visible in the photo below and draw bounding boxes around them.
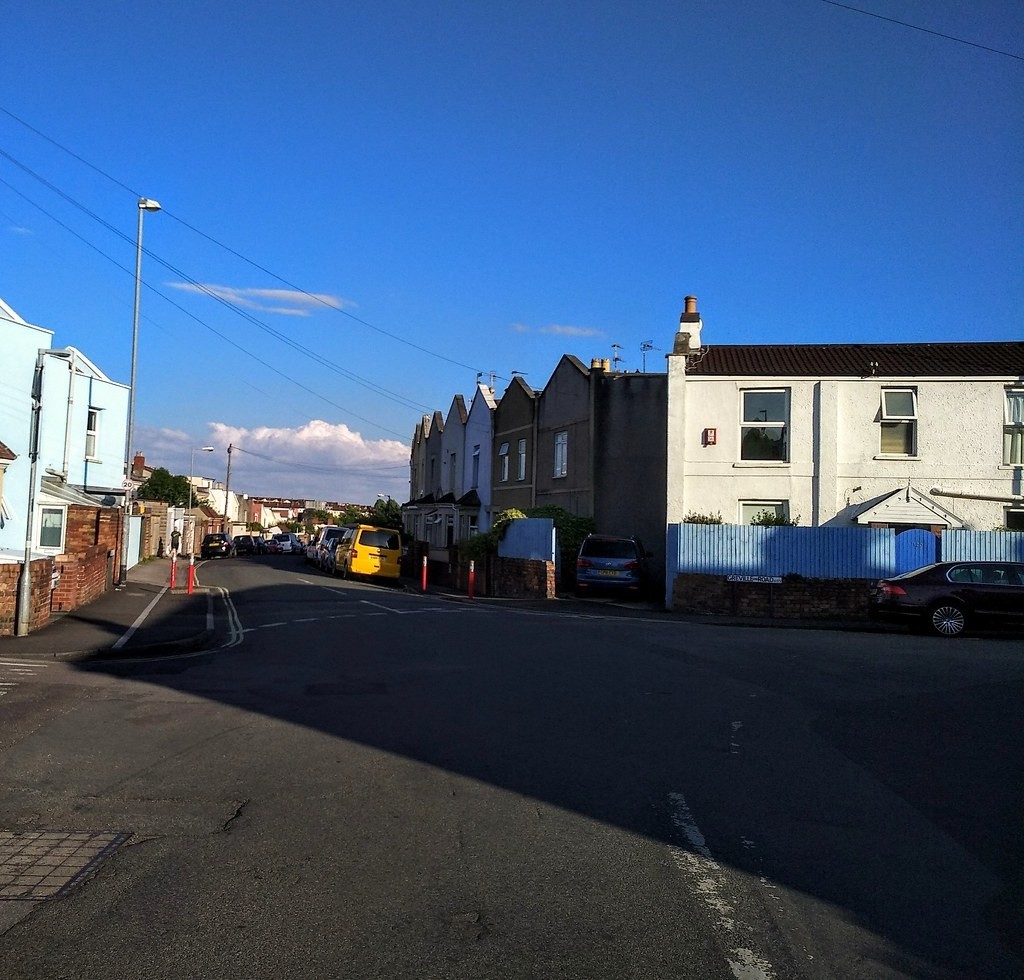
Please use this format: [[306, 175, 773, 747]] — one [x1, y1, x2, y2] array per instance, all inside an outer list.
[[331, 524, 402, 586], [313, 526, 350, 566]]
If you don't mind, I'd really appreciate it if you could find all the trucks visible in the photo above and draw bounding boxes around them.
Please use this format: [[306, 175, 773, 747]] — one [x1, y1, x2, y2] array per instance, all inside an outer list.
[[272, 533, 301, 554]]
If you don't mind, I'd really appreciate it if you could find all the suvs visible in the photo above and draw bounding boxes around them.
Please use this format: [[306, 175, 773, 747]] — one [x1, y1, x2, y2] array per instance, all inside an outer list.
[[576, 533, 654, 598], [200, 532, 236, 560]]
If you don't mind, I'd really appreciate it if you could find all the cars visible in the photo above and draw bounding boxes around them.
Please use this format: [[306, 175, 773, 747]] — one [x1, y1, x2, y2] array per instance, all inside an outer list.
[[320, 538, 342, 574], [307, 540, 318, 561], [870, 561, 1024, 638], [233, 535, 256, 556], [253, 536, 269, 556], [265, 540, 283, 554]]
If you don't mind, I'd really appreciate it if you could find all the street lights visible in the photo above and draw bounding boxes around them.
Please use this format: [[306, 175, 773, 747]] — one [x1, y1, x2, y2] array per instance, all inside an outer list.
[[119, 197, 162, 588], [186, 446, 215, 559]]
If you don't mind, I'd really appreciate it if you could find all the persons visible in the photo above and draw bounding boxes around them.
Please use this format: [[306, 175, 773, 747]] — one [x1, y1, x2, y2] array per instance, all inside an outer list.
[[171, 527, 181, 553]]
